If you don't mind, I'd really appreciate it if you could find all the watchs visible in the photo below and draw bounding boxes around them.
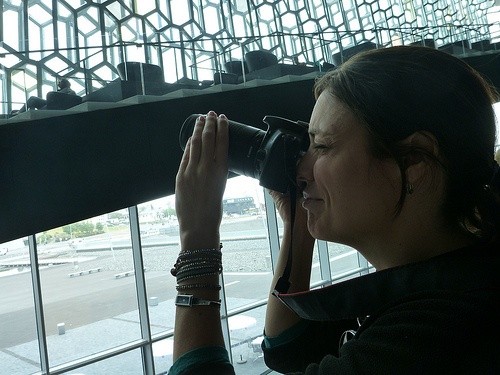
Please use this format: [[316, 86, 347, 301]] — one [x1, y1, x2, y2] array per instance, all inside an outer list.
[[175, 295, 222, 308]]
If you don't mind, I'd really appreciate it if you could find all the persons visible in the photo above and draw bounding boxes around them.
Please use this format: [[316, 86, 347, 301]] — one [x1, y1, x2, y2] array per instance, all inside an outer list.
[[170, 45, 500, 375]]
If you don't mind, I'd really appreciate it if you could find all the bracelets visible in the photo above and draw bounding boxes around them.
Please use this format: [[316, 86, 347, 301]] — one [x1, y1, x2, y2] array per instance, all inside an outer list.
[[171, 243, 222, 289]]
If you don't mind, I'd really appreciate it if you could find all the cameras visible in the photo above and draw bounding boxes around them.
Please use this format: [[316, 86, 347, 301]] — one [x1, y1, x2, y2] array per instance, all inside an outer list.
[[179, 115, 310, 195]]
